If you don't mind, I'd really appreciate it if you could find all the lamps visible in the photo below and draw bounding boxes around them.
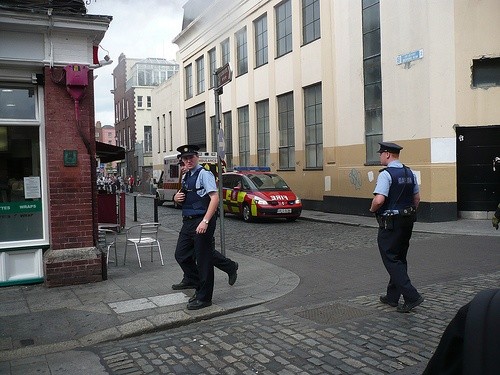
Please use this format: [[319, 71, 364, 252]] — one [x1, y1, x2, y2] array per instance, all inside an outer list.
[[99, 45, 110, 61]]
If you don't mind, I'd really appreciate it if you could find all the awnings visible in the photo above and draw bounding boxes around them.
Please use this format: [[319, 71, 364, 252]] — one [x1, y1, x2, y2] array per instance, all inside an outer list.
[[96, 141, 125, 163]]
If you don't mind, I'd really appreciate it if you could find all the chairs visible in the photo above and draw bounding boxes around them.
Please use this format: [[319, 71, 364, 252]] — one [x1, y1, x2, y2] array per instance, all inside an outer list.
[[123, 223, 165, 269], [98, 225, 118, 269]]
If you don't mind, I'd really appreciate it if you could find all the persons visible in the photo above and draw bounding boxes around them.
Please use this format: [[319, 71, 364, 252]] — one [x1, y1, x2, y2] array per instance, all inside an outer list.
[[492, 203, 500, 230], [172, 144, 238, 310], [370, 143, 424, 312], [97, 175, 142, 193]]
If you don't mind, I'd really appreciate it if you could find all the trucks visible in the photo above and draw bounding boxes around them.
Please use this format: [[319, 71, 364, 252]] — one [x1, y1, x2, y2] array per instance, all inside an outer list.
[[152, 151, 221, 209]]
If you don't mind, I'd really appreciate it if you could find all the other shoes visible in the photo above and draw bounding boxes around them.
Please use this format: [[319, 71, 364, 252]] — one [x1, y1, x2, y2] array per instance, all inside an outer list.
[[188, 290, 199, 302], [397, 294, 424, 312], [187, 298, 212, 310], [228, 262, 238, 285], [172, 280, 198, 289], [380, 294, 398, 307]]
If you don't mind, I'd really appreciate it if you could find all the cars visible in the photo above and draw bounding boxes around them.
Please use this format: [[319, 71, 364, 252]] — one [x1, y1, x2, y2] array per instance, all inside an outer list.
[[216, 165, 304, 223]]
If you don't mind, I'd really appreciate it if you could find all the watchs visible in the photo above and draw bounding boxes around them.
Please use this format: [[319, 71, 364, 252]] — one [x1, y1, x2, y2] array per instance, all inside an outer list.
[[203, 220, 209, 224]]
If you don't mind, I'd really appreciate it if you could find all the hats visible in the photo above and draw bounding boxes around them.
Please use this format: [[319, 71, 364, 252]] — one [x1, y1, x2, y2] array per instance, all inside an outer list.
[[176, 155, 183, 164], [376, 142, 403, 153], [177, 145, 200, 157]]
